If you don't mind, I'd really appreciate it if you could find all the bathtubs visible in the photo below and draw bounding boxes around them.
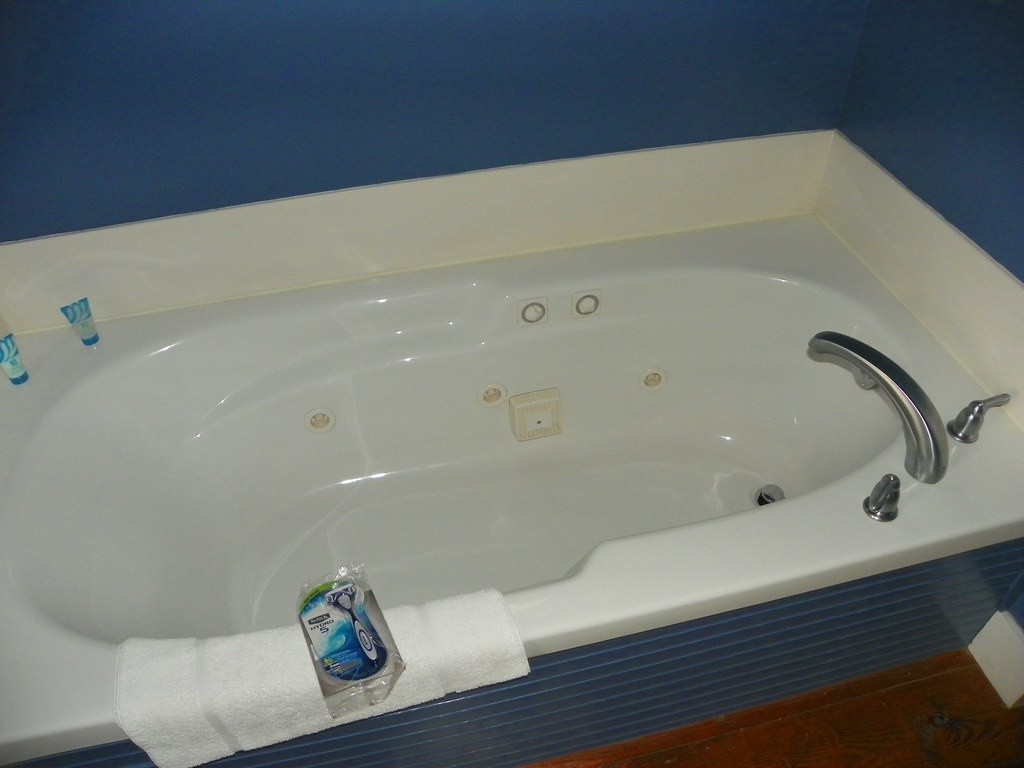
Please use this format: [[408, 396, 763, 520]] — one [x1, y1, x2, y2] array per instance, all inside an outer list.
[[1, 214, 1024, 768]]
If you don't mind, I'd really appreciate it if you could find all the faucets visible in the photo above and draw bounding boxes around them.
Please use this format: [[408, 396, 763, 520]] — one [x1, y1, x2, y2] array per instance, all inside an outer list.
[[810, 329, 950, 486]]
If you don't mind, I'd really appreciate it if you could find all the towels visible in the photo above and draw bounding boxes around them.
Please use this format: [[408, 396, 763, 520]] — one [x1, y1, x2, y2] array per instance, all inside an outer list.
[[109, 587, 532, 768]]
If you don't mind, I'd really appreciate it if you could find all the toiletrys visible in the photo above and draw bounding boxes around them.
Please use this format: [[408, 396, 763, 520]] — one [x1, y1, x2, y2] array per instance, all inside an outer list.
[[2, 333, 30, 385], [60, 294, 102, 346]]
